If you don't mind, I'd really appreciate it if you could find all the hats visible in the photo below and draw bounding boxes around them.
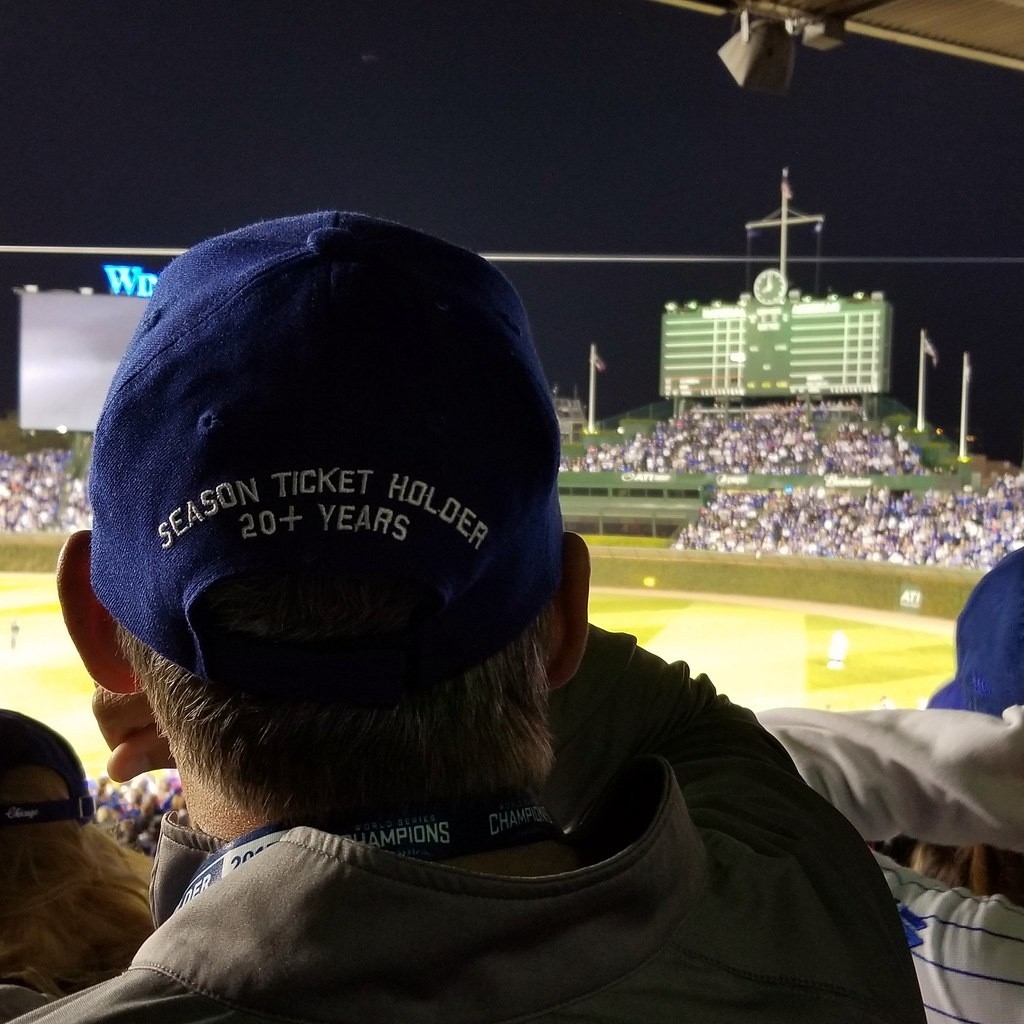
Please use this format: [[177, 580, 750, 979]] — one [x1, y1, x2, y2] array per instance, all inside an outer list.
[[87, 208, 561, 714], [0, 708, 94, 828], [924, 546, 1024, 719]]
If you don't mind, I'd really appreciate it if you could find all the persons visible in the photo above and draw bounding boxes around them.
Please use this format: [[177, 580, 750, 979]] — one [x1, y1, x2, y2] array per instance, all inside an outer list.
[[16, 205, 926, 1024], [566, 399, 921, 482], [1, 706, 159, 1022], [873, 549, 1024, 1023], [676, 470, 1024, 567]]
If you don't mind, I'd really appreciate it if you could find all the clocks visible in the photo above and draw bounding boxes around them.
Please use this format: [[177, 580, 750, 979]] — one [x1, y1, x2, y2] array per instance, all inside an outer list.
[[754, 271, 788, 304]]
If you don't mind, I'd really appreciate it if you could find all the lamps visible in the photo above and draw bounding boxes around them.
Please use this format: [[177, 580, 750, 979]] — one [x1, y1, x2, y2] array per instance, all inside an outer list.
[[715, 6, 799, 96]]
